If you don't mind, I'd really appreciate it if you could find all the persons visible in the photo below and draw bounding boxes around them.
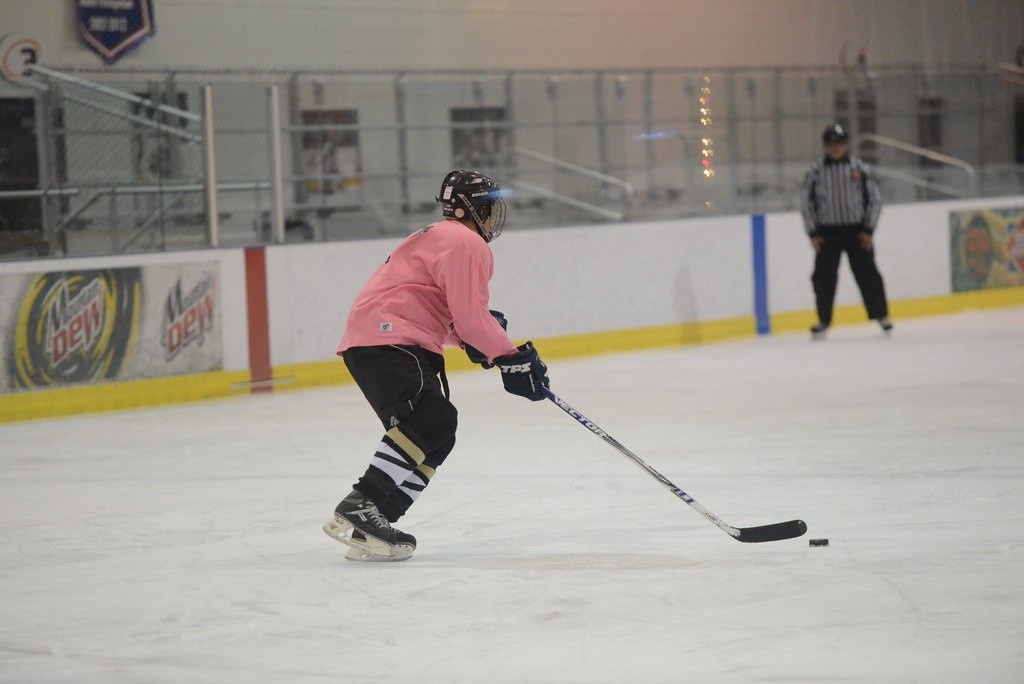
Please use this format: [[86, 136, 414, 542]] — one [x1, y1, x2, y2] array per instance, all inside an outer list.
[[800, 122, 893, 339], [321, 171, 550, 561]]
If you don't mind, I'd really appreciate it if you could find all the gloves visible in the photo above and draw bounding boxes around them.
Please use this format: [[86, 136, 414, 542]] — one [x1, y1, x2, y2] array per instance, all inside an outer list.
[[463, 309, 508, 369], [493, 341, 550, 401]]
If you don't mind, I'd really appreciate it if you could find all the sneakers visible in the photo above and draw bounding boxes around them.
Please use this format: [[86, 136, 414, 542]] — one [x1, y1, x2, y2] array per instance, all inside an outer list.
[[809, 322, 833, 339], [322, 490, 417, 562], [877, 317, 894, 337]]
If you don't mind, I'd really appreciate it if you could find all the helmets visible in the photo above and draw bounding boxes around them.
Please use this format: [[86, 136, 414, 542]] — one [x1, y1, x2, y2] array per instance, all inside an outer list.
[[821, 123, 850, 142], [435, 169, 507, 243]]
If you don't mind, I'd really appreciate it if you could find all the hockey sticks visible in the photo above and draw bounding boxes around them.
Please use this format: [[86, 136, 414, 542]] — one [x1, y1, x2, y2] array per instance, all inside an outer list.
[[540, 384, 807, 544]]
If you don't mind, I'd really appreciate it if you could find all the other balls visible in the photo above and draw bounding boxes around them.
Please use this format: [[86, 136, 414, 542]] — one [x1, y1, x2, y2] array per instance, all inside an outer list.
[[808, 537, 831, 547]]
